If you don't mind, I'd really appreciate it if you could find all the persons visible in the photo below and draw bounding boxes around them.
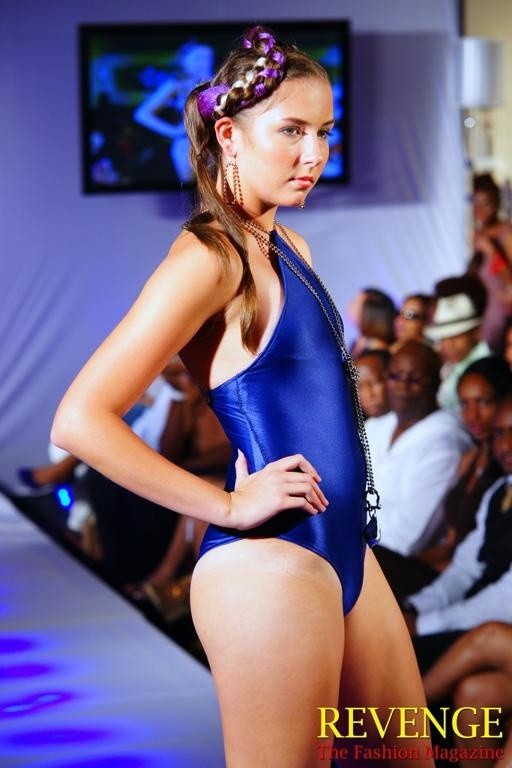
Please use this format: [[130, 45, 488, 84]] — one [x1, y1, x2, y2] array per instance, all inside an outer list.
[[18, 355, 238, 624], [341, 174, 512, 768], [133, 42, 211, 187], [47, 26, 436, 768]]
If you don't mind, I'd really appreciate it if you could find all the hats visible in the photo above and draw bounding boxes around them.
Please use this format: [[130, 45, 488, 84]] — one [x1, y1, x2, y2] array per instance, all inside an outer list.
[[422, 295, 484, 342]]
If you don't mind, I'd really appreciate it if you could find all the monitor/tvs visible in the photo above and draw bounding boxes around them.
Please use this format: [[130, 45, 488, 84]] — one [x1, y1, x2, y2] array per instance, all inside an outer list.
[[76, 18, 351, 195]]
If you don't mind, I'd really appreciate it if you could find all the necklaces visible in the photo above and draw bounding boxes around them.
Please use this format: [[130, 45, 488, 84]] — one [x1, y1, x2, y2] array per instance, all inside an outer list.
[[227, 209, 381, 548]]
